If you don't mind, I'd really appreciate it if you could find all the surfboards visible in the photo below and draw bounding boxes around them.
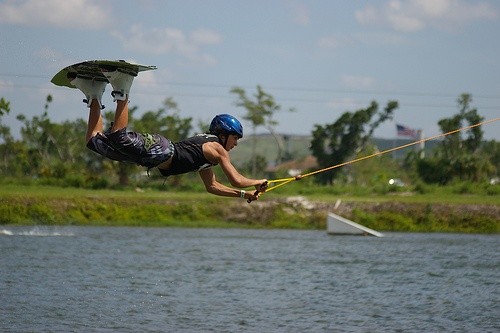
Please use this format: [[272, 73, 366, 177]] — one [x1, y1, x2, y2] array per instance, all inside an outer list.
[[50, 60, 158, 89]]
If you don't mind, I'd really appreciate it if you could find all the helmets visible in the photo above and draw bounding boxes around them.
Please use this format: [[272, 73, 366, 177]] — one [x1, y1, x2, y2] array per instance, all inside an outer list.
[[209, 113, 243, 138]]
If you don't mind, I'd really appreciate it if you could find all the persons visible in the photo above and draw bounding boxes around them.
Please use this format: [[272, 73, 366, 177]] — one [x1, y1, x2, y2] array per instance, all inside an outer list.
[[69, 70, 269, 200]]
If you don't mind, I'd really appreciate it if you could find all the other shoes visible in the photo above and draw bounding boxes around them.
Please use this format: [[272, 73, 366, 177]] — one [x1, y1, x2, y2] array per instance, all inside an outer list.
[[100, 66, 138, 102], [67, 73, 109, 110]]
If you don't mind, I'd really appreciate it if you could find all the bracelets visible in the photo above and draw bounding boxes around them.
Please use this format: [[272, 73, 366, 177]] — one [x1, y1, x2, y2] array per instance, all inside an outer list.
[[240, 189, 245, 199]]
